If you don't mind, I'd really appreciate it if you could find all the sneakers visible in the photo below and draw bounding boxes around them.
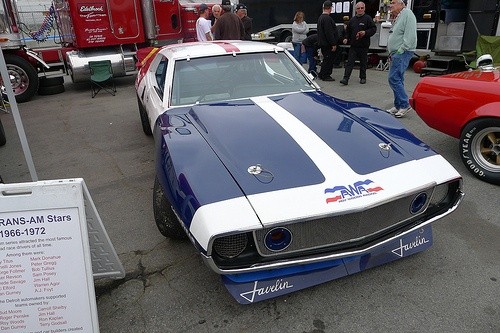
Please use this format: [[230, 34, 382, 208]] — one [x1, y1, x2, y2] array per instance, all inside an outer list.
[[395, 106, 411, 117], [385, 106, 398, 114]]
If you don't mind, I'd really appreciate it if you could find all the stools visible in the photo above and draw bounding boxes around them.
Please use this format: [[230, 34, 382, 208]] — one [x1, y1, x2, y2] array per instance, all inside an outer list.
[[375, 56, 391, 72]]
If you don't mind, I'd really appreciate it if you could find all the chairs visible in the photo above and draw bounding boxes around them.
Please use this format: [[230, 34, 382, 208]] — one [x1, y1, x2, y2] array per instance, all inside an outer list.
[[87, 59, 117, 99], [457, 35, 500, 71]]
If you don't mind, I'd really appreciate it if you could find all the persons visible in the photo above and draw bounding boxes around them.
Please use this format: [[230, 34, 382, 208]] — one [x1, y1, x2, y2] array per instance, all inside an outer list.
[[339, 2, 378, 85], [302, 33, 321, 80], [386, 0, 419, 118], [333, 20, 350, 68], [214, 4, 245, 40], [292, 11, 310, 63], [196, 3, 213, 42], [236, 3, 252, 41], [317, 0, 339, 81], [207, 4, 223, 40]]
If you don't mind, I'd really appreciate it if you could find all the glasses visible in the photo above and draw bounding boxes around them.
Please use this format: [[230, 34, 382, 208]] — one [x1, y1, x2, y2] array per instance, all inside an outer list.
[[357, 7, 364, 10]]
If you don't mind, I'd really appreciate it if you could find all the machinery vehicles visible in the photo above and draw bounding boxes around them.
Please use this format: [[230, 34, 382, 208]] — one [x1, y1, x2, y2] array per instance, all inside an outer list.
[[0, 0, 241, 104]]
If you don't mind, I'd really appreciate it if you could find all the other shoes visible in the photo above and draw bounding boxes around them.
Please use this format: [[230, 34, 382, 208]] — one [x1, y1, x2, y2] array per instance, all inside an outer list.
[[360, 78, 366, 83], [322, 76, 335, 81], [340, 78, 348, 85]]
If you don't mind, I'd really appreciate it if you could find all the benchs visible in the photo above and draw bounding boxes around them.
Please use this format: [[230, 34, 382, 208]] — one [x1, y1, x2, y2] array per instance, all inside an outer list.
[[177, 66, 245, 89]]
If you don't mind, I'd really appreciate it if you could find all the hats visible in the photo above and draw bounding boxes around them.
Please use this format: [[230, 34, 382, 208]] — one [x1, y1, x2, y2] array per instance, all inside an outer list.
[[199, 3, 208, 11], [323, 1, 332, 6], [235, 4, 247, 12]]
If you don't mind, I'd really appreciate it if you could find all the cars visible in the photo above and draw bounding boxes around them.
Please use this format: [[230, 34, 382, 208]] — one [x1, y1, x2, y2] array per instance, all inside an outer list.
[[133, 39, 466, 305], [409, 67, 500, 186]]
